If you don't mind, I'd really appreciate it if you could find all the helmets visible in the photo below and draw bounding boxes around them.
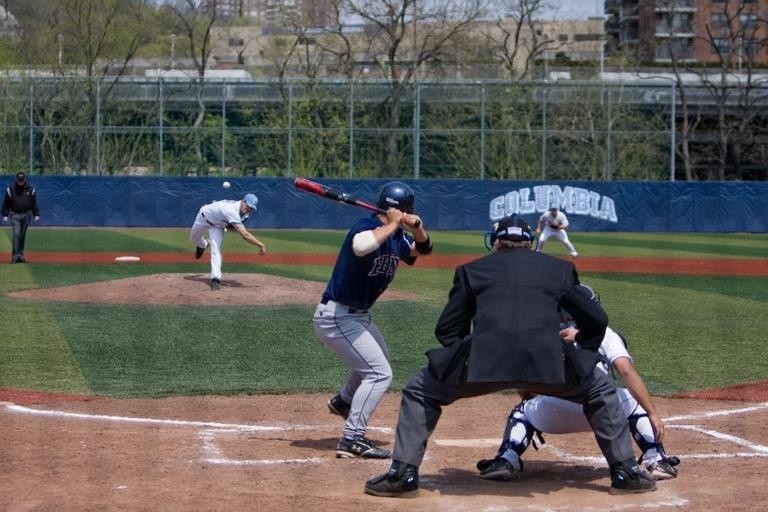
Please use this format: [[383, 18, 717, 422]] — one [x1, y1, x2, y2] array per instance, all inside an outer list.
[[485, 212, 539, 251], [377, 182, 414, 214]]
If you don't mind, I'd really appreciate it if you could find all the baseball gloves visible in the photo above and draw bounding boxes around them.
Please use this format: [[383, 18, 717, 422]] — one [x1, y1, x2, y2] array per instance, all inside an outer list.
[[548, 218, 560, 230]]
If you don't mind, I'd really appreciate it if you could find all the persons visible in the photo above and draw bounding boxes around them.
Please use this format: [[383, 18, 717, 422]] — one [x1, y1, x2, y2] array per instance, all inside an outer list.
[[476, 283, 679, 480], [1, 172, 40, 261], [312, 182, 433, 458], [191, 194, 267, 290], [364, 214, 657, 497], [536, 202, 578, 257]]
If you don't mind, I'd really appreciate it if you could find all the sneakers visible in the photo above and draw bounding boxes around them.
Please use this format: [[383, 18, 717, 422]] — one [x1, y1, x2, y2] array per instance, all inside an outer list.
[[327, 391, 351, 421], [336, 437, 391, 458], [196, 240, 210, 259], [211, 278, 221, 289], [477, 459, 514, 481], [609, 457, 657, 493], [365, 460, 420, 498], [571, 252, 578, 256], [639, 457, 678, 479], [19, 256, 26, 262], [12, 258, 20, 263]]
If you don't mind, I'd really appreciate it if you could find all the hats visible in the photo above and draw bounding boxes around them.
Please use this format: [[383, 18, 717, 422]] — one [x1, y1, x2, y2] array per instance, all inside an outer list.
[[17, 172, 25, 178], [551, 203, 558, 208], [243, 194, 258, 210]]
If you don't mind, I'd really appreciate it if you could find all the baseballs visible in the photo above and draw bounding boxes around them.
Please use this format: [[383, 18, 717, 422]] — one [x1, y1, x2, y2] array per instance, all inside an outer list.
[[223, 182, 230, 187]]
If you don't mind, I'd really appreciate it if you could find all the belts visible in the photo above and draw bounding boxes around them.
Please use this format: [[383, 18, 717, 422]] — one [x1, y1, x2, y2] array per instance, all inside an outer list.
[[320, 297, 369, 313], [201, 213, 215, 227]]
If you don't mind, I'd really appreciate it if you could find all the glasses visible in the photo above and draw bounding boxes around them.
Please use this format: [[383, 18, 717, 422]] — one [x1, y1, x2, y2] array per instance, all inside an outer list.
[[19, 179, 25, 181]]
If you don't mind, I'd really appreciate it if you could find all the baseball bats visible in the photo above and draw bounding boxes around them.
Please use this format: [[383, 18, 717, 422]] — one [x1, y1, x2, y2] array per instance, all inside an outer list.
[[294, 176, 420, 228]]
